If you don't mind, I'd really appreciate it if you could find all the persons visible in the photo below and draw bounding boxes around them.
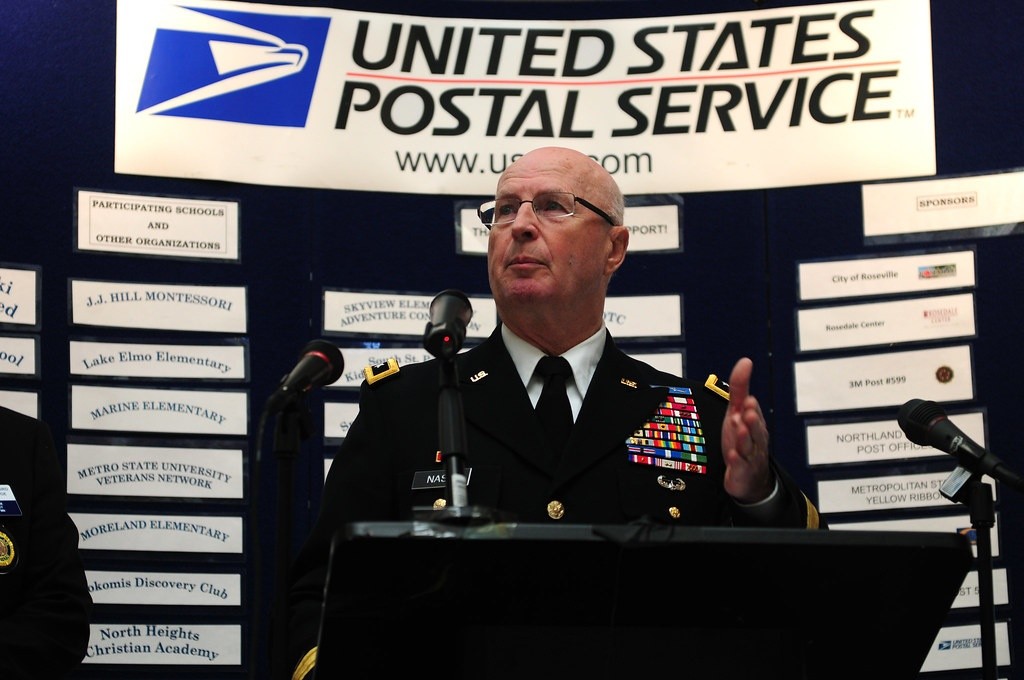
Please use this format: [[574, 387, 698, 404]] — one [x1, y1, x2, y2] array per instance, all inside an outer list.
[[0, 407, 98, 680], [280, 147, 830, 680]]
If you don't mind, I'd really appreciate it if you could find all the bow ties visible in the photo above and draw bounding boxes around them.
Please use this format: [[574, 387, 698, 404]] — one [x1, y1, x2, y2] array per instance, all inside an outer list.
[[529, 356, 576, 461]]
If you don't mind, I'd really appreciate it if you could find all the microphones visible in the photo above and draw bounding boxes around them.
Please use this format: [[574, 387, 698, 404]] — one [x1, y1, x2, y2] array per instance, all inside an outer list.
[[421, 290, 496, 525], [896, 397, 1023, 492], [262, 339, 344, 425]]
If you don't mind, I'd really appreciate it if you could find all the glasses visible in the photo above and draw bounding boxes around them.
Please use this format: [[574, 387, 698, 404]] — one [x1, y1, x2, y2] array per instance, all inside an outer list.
[[477, 193, 622, 233]]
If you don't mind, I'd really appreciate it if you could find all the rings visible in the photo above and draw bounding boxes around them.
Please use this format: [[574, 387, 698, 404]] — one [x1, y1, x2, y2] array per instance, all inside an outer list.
[[741, 441, 758, 463]]
